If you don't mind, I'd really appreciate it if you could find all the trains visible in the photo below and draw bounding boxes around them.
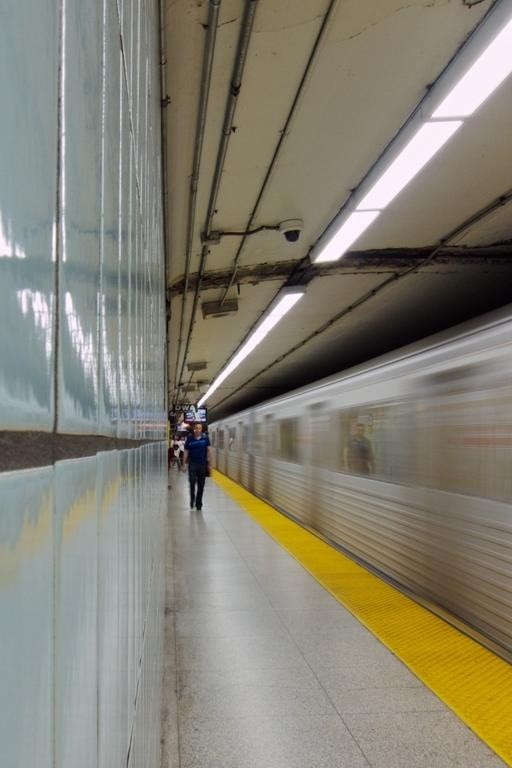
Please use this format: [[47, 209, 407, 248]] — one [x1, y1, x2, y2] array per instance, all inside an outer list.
[[203, 303, 512, 655]]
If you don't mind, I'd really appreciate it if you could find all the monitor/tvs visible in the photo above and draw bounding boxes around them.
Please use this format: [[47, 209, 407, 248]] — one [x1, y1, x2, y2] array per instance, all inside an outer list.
[[185, 408, 206, 423]]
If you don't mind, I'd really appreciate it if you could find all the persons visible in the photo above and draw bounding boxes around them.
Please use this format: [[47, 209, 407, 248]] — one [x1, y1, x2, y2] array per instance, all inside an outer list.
[[184, 420, 214, 511], [168, 430, 186, 473], [343, 421, 377, 476]]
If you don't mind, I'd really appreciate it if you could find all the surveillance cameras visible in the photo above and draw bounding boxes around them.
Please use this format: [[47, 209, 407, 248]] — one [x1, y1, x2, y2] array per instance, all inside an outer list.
[[281, 220, 304, 242]]
